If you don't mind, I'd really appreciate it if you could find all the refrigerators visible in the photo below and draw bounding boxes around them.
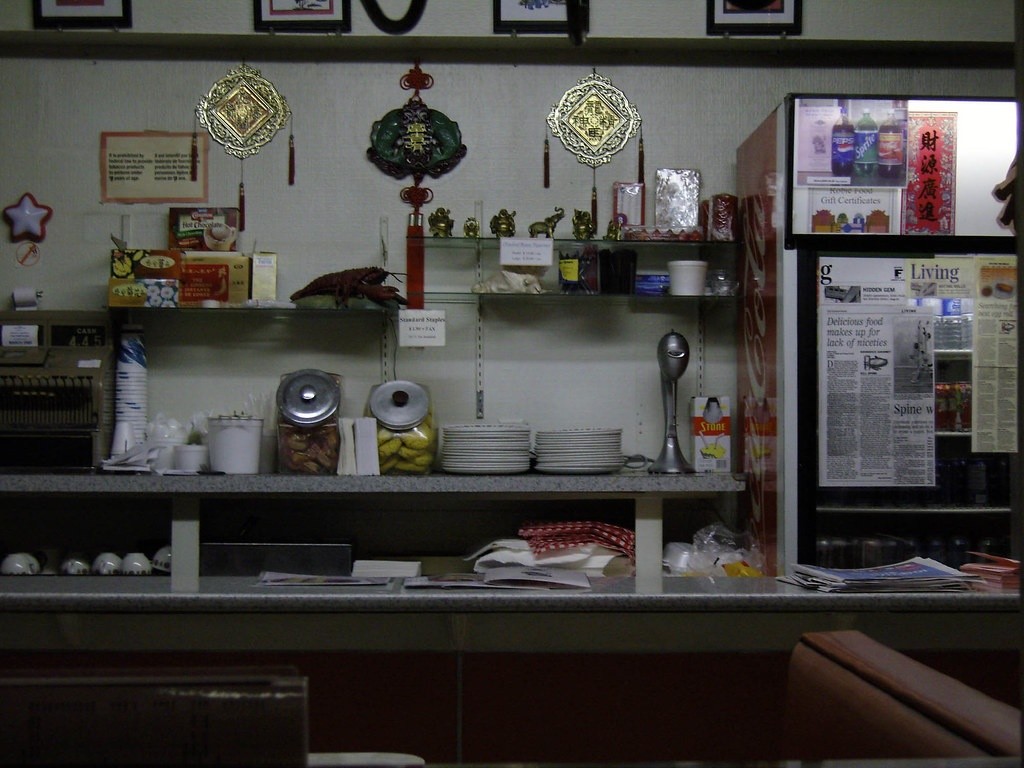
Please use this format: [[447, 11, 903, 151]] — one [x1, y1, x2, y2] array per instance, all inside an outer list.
[[736, 93, 1024, 578]]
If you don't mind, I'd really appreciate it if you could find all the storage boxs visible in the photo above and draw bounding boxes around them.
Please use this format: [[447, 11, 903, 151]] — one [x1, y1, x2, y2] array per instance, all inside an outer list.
[[690, 394, 731, 475], [109, 205, 278, 307]]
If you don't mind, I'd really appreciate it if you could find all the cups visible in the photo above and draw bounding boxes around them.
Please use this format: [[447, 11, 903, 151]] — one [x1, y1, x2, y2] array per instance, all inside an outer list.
[[203, 223, 236, 252], [110, 422, 136, 455], [115, 323, 148, 446]]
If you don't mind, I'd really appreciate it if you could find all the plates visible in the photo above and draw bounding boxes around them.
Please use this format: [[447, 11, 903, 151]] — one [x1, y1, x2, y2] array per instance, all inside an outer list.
[[440, 421, 531, 475], [532, 427, 627, 475]]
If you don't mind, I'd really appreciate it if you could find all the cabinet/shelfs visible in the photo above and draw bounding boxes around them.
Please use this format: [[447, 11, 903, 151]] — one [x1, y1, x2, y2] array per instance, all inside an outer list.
[[0, 234, 749, 580]]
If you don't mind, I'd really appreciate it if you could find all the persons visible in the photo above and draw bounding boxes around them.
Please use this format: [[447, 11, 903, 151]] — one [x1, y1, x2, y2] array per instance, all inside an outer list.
[[428, 207, 455, 237], [489, 209, 516, 238], [572, 212, 596, 240]]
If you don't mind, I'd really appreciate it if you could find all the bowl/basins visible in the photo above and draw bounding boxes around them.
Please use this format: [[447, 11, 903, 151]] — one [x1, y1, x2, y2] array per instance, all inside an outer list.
[[120, 552, 152, 576], [90, 552, 122, 576], [1, 552, 40, 576], [174, 446, 208, 472], [152, 544, 171, 573], [60, 553, 90, 575]]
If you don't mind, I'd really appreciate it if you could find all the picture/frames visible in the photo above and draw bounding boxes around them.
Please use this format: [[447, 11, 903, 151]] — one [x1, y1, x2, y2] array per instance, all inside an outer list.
[[253, 0, 352, 33], [705, 0, 804, 37], [32, 0, 132, 30], [492, 0, 590, 33]]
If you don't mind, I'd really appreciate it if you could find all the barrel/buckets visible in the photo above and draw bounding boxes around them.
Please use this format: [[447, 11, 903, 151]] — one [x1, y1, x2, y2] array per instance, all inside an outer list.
[[207, 419, 263, 475], [669, 261, 708, 295]]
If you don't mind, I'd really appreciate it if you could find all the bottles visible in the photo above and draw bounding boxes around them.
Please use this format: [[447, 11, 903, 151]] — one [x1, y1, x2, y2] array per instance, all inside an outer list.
[[854, 108, 879, 178], [832, 108, 854, 176], [879, 109, 903, 178]]
